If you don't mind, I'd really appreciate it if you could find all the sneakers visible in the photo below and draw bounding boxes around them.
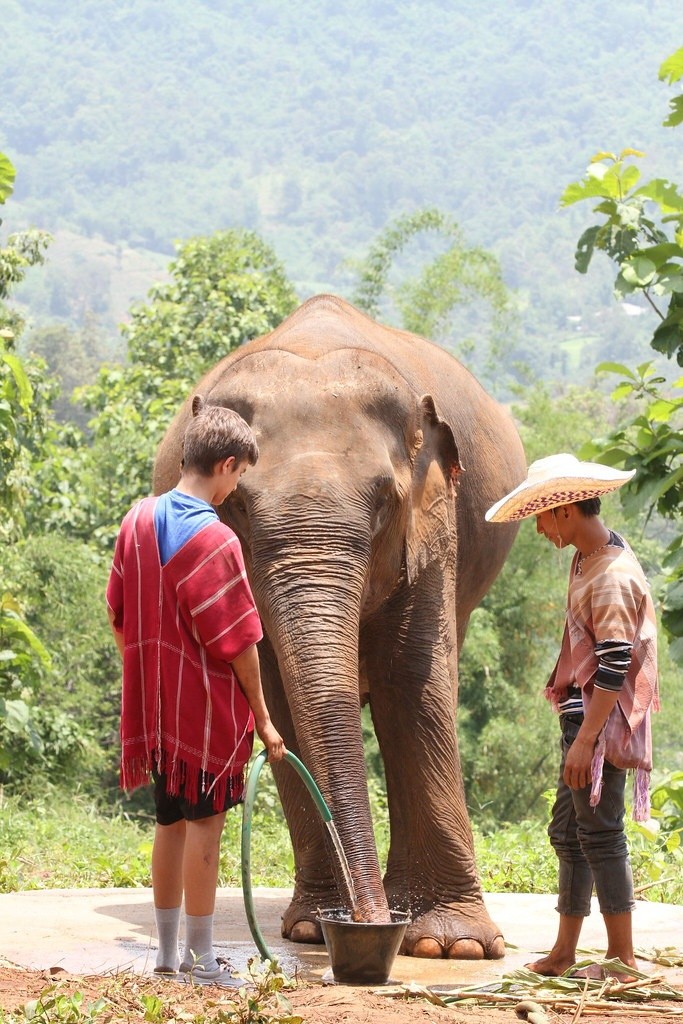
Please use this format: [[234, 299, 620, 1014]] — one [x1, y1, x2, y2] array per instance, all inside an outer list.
[[152, 966, 181, 976], [174, 955, 257, 992]]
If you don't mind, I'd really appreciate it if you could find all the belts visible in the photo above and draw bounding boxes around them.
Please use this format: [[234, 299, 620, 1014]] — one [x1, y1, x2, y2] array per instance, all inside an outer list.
[[565, 712, 584, 726]]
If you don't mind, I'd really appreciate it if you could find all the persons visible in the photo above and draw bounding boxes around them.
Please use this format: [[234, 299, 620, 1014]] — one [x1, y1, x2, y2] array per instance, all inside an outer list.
[[486, 453, 657, 981], [105, 406, 286, 986]]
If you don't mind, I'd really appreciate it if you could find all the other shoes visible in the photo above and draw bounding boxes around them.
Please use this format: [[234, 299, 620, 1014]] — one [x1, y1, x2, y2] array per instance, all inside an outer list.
[[566, 960, 639, 983]]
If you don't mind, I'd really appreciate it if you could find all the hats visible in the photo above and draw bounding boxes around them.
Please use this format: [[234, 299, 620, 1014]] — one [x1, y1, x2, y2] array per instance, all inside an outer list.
[[485, 453, 637, 524]]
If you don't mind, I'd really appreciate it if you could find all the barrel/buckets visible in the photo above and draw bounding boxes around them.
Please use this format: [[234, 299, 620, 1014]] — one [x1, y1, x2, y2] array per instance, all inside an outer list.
[[310, 908, 412, 984]]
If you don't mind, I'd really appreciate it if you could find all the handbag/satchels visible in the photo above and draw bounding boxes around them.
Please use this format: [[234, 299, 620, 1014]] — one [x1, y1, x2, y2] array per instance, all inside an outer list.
[[589, 700, 653, 822]]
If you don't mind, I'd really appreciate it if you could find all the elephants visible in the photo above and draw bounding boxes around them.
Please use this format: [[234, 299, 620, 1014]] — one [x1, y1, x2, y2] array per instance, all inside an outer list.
[[152, 294, 527, 959]]
